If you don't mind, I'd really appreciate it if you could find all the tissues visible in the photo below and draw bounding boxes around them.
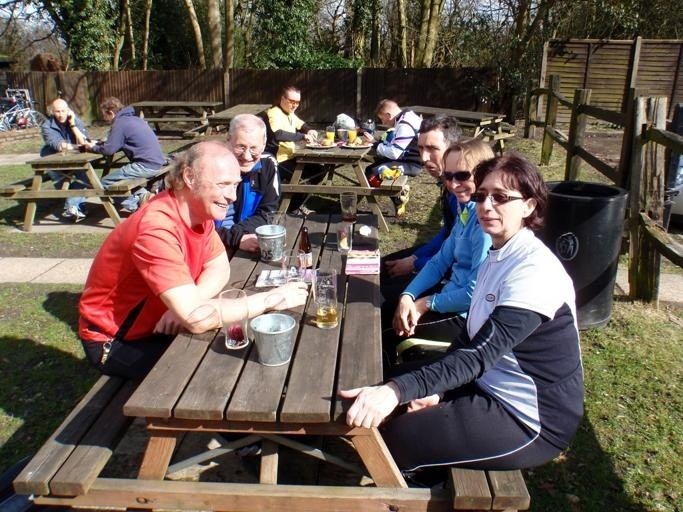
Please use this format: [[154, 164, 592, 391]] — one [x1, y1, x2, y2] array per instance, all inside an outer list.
[[352, 211, 380, 250], [332, 113, 356, 139]]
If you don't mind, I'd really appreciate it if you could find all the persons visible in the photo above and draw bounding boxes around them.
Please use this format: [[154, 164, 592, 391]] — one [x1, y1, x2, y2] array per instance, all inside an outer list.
[[76, 143, 310, 483], [339, 99, 423, 218], [40, 99, 92, 224], [379, 111, 464, 300], [84, 95, 167, 214], [314, 155, 585, 488], [212, 113, 281, 264], [382, 138, 495, 370], [257, 85, 326, 212]]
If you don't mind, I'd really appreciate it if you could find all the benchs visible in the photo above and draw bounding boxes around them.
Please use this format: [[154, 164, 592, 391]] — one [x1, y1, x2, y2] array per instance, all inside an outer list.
[[183, 103, 272, 138], [12, 372, 146, 511], [128, 100, 224, 139], [0, 146, 177, 233], [280, 131, 408, 233], [445, 466, 531, 512], [377, 106, 516, 150]]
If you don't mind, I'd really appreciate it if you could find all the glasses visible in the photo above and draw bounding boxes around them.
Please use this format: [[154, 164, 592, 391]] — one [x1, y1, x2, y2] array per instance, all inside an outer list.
[[444, 172, 470, 180], [471, 193, 524, 203]]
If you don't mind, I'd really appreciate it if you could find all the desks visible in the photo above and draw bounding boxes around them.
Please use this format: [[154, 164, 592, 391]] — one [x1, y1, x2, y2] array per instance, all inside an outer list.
[[122, 214, 408, 512]]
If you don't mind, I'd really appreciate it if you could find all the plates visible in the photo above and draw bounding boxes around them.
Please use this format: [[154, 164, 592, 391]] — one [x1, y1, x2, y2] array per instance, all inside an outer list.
[[342, 141, 374, 148], [306, 142, 336, 148]]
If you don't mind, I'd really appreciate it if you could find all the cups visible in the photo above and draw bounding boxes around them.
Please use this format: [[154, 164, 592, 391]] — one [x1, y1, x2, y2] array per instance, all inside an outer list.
[[267, 210, 287, 247], [326, 126, 335, 143], [336, 129, 348, 142], [59, 139, 68, 157], [340, 190, 358, 224], [310, 266, 339, 330], [349, 127, 357, 143], [336, 221, 353, 254], [282, 247, 305, 282], [216, 289, 250, 352]]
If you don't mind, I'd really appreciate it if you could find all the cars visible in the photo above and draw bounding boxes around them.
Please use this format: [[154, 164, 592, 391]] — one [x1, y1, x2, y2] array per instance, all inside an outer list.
[[669, 154, 683, 216]]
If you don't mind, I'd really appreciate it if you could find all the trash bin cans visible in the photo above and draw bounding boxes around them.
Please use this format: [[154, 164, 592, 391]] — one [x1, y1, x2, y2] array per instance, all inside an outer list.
[[538, 180, 630, 330]]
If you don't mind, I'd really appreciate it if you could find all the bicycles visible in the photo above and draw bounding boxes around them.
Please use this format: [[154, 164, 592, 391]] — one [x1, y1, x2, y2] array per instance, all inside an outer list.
[[0, 96, 48, 132]]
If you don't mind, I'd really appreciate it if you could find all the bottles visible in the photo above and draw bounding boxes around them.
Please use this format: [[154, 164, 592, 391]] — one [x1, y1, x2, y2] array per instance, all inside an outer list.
[[297, 227, 314, 270], [366, 119, 373, 136]]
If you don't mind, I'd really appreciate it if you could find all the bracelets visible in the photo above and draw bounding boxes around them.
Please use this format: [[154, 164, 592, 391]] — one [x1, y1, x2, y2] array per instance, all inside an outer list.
[[70, 124, 76, 128], [425, 295, 432, 310]]
